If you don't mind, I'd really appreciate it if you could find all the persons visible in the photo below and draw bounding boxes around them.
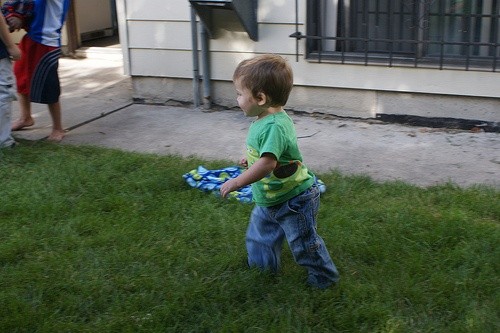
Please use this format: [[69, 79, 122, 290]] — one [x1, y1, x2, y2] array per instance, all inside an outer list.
[[3, 0, 68, 143], [0, 0, 24, 149], [218, 54, 341, 289]]
